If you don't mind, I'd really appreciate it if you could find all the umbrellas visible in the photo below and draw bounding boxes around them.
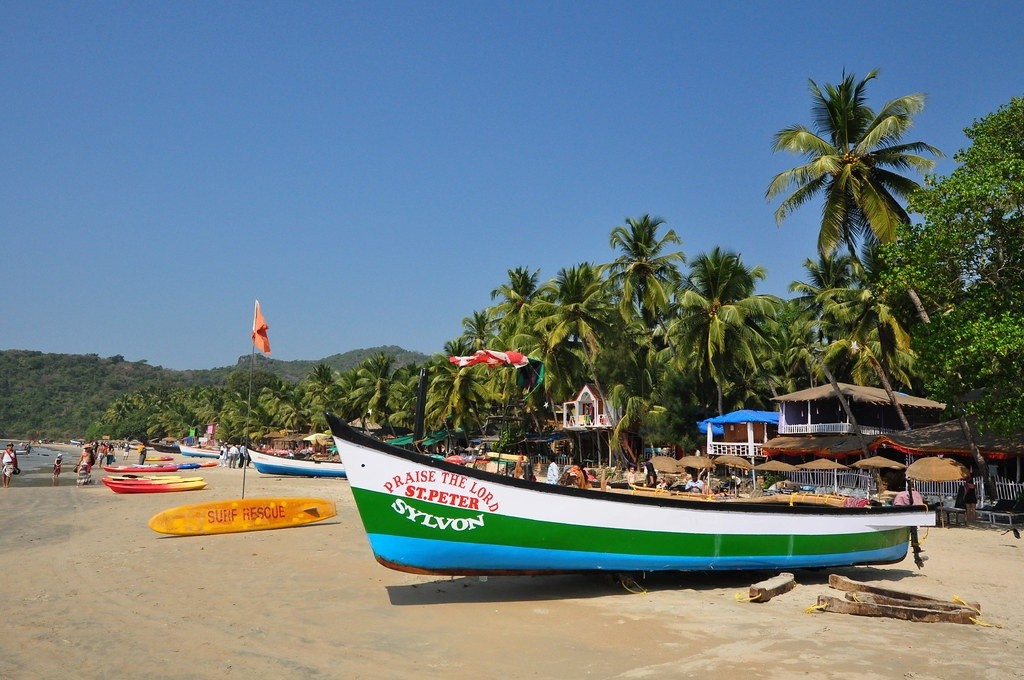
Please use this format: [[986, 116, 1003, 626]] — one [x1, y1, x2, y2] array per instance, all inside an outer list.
[[304, 433, 330, 440], [754, 456, 969, 527], [714, 456, 752, 498], [348, 415, 382, 433], [651, 455, 716, 478]]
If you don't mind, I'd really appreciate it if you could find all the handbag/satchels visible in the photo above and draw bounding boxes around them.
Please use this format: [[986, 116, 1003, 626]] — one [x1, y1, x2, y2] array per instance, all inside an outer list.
[[11, 457, 18, 466], [72, 465, 79, 474]]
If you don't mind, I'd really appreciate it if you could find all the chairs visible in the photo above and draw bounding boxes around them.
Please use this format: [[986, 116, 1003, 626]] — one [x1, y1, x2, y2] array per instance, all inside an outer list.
[[936, 498, 1024, 528]]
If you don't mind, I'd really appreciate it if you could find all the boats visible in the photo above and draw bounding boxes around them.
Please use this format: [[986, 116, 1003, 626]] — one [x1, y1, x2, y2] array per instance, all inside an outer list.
[[178, 444, 241, 459], [321, 408, 935, 578], [69, 439, 85, 445], [247, 449, 346, 478], [124, 442, 154, 450], [0, 449, 27, 455], [149, 441, 181, 453]]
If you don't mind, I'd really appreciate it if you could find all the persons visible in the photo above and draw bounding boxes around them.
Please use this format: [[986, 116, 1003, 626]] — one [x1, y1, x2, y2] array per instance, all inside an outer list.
[[197, 442, 202, 448], [645, 462, 656, 487], [18, 442, 31, 454], [219, 442, 252, 468], [685, 474, 704, 493], [657, 479, 667, 489], [573, 461, 592, 488], [964, 477, 977, 526], [585, 404, 593, 415], [547, 453, 559, 485], [727, 473, 742, 496], [53, 454, 63, 477], [627, 467, 637, 488], [0, 442, 18, 488], [118, 442, 130, 460], [139, 444, 146, 464], [76, 441, 116, 487]]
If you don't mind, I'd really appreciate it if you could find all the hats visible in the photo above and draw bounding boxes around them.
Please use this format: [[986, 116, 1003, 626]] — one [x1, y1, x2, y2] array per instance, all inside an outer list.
[[83, 444, 94, 449], [6, 442, 14, 446], [57, 453, 63, 458]]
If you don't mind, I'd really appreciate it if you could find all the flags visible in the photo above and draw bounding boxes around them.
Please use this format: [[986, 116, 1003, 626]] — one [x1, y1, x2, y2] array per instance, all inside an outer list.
[[252, 302, 270, 355]]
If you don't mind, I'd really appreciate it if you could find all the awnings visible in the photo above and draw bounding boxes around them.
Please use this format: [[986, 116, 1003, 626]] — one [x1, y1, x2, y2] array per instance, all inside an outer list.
[[388, 436, 442, 451]]
